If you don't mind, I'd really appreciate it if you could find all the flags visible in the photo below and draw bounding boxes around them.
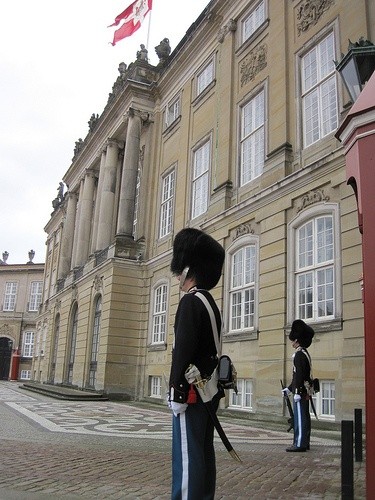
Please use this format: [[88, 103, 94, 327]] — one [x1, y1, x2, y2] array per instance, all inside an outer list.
[[107, 0, 152, 49]]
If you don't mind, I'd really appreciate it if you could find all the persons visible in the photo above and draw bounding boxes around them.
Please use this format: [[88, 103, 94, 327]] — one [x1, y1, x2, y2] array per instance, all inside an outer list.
[[280, 319, 315, 452], [167, 228, 226, 500]]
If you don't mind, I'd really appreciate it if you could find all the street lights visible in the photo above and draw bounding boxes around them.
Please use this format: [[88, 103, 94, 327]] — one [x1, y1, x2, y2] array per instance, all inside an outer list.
[[331, 35, 375, 104], [7, 339, 16, 351]]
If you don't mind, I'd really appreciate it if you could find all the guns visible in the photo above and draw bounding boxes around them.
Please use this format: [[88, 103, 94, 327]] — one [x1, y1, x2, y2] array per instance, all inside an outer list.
[[161, 370, 170, 403], [279, 379, 295, 445]]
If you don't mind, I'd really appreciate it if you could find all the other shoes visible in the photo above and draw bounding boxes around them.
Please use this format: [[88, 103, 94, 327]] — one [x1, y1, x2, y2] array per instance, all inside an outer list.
[[286, 445, 307, 452]]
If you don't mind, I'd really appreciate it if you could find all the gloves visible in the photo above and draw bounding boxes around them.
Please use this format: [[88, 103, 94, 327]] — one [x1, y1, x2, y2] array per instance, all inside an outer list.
[[281, 388, 291, 395], [171, 400, 188, 417], [294, 394, 301, 402]]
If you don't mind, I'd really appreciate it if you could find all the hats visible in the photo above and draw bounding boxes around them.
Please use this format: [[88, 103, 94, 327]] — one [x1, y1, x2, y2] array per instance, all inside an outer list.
[[171, 228, 225, 290], [288, 319, 314, 348]]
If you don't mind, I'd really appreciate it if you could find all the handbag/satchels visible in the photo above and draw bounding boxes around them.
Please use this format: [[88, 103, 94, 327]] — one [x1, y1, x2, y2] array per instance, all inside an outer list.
[[313, 378, 320, 393], [218, 355, 237, 389]]
